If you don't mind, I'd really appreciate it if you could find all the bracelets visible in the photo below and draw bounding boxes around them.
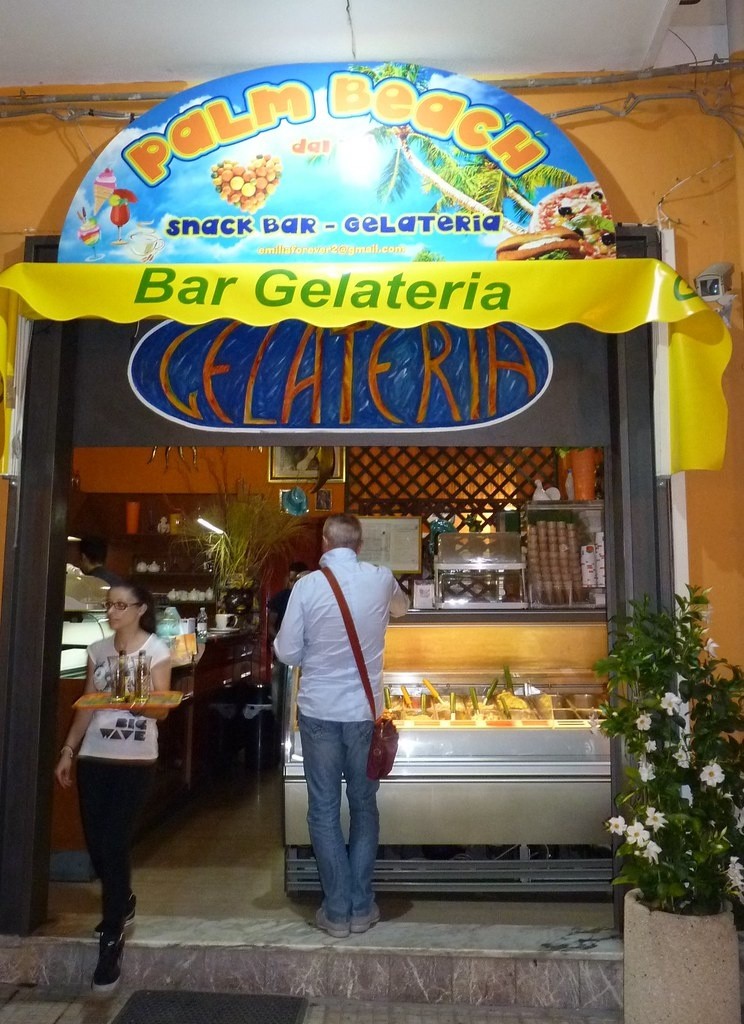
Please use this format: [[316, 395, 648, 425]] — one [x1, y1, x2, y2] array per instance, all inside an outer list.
[[60, 745, 74, 758], [133, 711, 144, 718]]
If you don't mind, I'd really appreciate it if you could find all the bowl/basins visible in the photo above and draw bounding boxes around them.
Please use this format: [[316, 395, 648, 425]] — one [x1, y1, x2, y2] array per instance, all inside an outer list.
[[580, 531, 605, 588]]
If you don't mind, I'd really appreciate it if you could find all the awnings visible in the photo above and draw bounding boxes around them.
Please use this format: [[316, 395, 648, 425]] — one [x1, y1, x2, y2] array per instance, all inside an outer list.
[[0, 261, 733, 486]]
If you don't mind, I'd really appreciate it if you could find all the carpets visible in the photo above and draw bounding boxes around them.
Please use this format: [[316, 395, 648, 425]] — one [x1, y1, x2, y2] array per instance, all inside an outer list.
[[110, 990, 308, 1023]]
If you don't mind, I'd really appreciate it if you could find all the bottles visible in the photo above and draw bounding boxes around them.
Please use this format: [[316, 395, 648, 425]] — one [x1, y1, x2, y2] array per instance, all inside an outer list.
[[170, 507, 182, 534], [135, 650, 150, 700], [115, 650, 131, 701], [196, 608, 208, 638], [565, 469, 575, 500]]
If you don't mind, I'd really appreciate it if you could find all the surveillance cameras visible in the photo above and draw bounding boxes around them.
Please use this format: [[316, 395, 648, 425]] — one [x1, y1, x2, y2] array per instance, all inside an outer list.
[[695, 261, 736, 302]]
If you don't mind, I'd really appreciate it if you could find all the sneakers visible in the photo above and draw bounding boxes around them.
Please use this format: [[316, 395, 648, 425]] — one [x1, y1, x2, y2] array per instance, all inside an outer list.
[[314, 908, 350, 937], [91, 930, 125, 992], [349, 900, 380, 933], [93, 895, 137, 937]]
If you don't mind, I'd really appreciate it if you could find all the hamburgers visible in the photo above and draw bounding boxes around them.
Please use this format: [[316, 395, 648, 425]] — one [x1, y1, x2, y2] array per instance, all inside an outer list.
[[495, 227, 585, 261]]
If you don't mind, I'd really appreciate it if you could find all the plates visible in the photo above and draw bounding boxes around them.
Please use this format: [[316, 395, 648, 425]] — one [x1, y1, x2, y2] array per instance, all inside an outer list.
[[210, 627, 240, 634]]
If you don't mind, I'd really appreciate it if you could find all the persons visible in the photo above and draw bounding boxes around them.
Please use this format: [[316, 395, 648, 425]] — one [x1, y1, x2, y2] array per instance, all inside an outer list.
[[272, 512, 410, 937], [54, 581, 172, 992], [263, 562, 308, 724], [79, 536, 124, 586]]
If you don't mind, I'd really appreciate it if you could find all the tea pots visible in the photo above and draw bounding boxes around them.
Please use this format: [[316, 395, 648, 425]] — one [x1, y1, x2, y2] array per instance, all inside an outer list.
[[166, 588, 213, 601], [136, 561, 161, 572]]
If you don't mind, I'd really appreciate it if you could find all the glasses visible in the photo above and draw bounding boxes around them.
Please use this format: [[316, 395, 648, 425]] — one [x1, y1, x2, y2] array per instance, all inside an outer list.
[[103, 600, 142, 611]]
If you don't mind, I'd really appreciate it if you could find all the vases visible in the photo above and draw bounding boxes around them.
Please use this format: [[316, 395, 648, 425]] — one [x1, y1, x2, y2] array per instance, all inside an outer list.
[[623, 887, 743, 1024]]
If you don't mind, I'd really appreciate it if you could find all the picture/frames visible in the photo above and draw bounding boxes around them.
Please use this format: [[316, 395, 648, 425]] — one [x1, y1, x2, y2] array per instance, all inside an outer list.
[[267, 446, 348, 482]]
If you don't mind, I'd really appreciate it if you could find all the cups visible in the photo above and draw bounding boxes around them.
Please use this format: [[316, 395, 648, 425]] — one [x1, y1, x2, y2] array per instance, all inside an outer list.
[[215, 613, 238, 628], [126, 501, 140, 534], [107, 656, 129, 702], [132, 657, 152, 700]]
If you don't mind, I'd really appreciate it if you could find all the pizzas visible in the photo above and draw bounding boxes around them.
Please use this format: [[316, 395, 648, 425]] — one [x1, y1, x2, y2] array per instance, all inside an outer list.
[[530, 180, 617, 259]]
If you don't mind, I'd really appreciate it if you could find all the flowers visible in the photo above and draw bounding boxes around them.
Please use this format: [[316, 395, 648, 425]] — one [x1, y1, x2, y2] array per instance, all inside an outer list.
[[591, 585, 744, 915]]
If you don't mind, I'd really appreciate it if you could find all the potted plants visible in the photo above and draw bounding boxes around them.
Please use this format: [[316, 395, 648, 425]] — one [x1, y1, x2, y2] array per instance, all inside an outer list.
[[178, 478, 300, 623]]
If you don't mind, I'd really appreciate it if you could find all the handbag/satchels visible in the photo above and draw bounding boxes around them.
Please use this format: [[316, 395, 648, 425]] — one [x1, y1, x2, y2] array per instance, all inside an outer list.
[[366, 716, 399, 779]]
